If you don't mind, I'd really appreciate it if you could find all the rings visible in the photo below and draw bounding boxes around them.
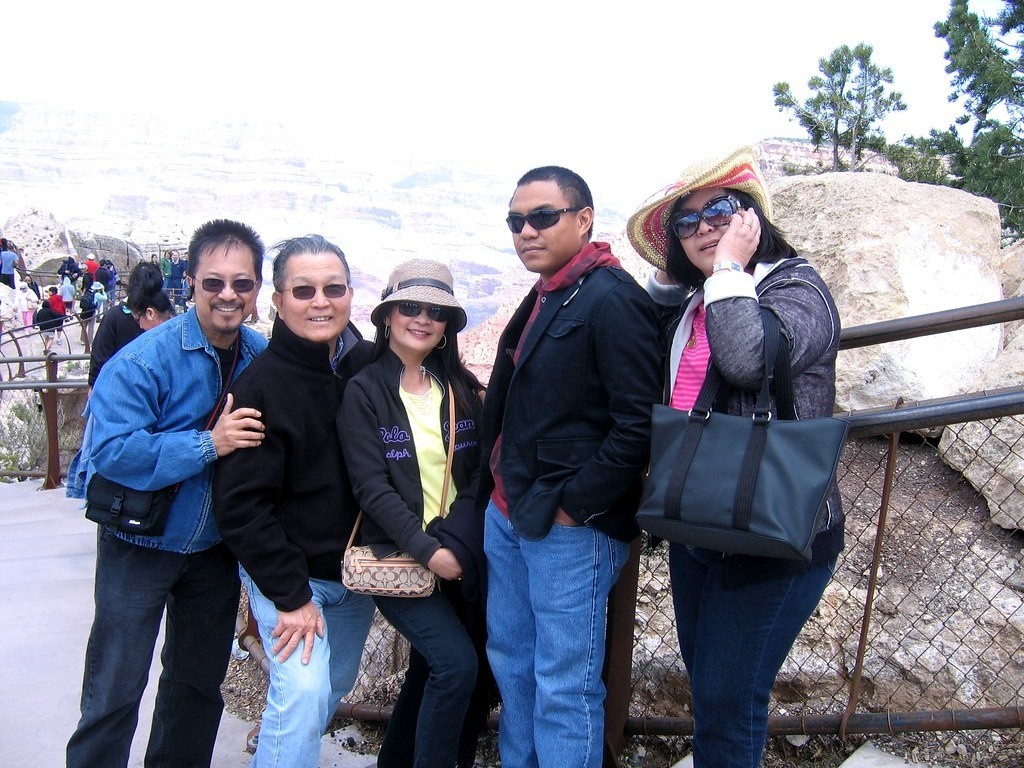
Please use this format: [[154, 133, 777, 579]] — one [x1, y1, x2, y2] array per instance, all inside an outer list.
[[742, 222, 753, 227], [457, 577, 462, 580]]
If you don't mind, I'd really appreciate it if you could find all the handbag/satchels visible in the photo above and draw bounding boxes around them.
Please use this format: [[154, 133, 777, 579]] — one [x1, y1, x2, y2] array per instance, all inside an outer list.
[[26, 289, 38, 310], [341, 542, 437, 597], [637, 305, 852, 560], [85, 472, 179, 535]]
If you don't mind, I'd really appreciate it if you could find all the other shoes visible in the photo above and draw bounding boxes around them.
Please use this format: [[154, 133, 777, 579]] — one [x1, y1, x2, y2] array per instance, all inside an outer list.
[[35, 325, 39, 329], [43, 349, 50, 355], [57, 339, 61, 345], [25, 331, 31, 336], [96, 318, 102, 323], [65, 319, 69, 322], [70, 316, 73, 320]]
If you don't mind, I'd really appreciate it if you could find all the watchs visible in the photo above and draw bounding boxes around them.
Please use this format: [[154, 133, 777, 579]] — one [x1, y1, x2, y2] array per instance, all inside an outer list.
[[713, 260, 743, 272]]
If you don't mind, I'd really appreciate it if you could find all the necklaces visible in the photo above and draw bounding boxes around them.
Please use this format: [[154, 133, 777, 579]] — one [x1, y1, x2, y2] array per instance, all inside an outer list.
[[687, 304, 706, 348]]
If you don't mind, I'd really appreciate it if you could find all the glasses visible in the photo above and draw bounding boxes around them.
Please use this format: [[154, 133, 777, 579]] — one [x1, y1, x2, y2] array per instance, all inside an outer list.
[[135, 306, 152, 325], [506, 206, 584, 234], [670, 195, 745, 239], [19, 288, 26, 290], [284, 282, 352, 300], [393, 301, 451, 322], [190, 274, 258, 293]]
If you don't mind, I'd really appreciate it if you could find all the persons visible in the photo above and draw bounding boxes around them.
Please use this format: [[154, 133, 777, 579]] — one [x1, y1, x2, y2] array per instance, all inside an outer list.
[[626, 146, 846, 768], [0, 237, 194, 394], [65, 165, 666, 768]]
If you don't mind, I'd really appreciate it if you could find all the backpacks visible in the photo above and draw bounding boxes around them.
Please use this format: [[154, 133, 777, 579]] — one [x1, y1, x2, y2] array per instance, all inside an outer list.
[[79, 290, 100, 310]]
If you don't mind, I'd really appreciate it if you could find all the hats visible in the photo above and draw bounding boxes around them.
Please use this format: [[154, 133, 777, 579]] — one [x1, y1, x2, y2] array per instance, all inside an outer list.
[[87, 253, 95, 259], [91, 281, 103, 290], [78, 264, 88, 268], [370, 259, 468, 334], [627, 145, 773, 274]]
